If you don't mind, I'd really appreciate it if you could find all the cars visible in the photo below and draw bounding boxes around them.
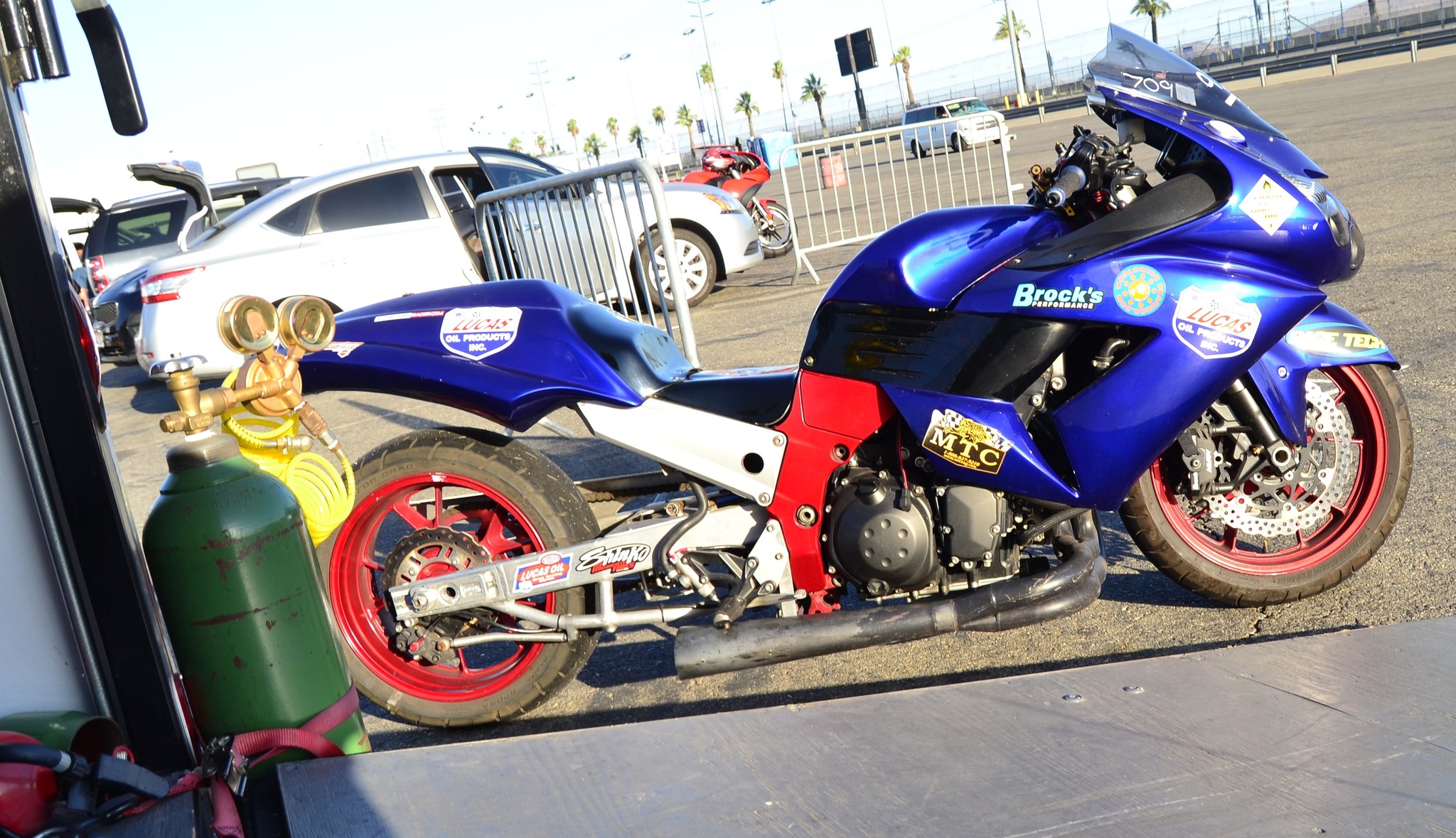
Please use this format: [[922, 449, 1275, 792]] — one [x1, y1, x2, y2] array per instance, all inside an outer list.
[[88, 263, 149, 362], [128, 145, 763, 382]]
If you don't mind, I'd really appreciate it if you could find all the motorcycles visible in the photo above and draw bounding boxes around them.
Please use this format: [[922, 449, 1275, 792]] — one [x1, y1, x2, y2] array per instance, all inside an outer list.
[[277, 27, 1417, 734], [650, 145, 798, 258]]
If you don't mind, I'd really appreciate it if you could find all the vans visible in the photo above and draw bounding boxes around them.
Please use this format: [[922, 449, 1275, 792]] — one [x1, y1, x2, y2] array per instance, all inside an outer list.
[[901, 97, 1007, 158]]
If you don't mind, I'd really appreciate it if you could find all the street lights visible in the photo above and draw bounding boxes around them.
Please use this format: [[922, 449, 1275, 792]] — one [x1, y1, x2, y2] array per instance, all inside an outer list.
[[762, 0, 788, 133], [681, 28, 715, 146], [688, 0, 729, 148]]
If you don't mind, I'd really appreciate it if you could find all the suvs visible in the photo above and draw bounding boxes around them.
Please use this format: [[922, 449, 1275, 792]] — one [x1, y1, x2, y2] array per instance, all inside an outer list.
[[46, 170, 315, 296]]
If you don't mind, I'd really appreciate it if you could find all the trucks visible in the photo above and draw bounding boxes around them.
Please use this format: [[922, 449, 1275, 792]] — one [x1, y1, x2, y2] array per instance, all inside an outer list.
[[0, 0, 246, 836]]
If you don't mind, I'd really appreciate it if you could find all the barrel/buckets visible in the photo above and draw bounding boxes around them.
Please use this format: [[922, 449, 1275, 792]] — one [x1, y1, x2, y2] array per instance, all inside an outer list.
[[819, 153, 847, 189]]
